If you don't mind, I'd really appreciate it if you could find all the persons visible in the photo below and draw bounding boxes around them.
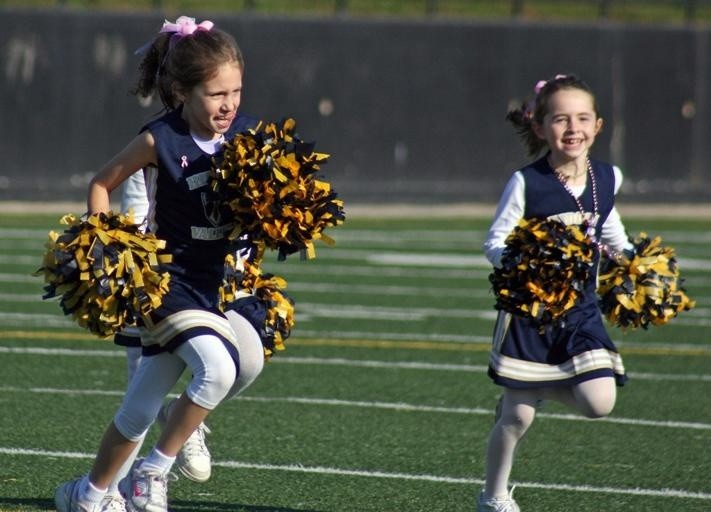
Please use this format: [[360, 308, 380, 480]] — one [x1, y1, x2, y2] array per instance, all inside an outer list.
[[120, 110, 264, 483], [53, 15, 246, 512], [475, 75, 638, 512]]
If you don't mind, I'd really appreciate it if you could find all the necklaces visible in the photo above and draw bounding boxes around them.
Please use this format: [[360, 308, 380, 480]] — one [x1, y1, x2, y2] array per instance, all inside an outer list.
[[550, 160, 601, 243]]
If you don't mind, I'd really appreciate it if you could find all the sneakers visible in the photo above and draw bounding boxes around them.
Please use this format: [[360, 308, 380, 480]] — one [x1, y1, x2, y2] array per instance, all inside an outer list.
[[476, 488, 521, 512], [159, 399, 213, 482], [53, 457, 169, 511]]
